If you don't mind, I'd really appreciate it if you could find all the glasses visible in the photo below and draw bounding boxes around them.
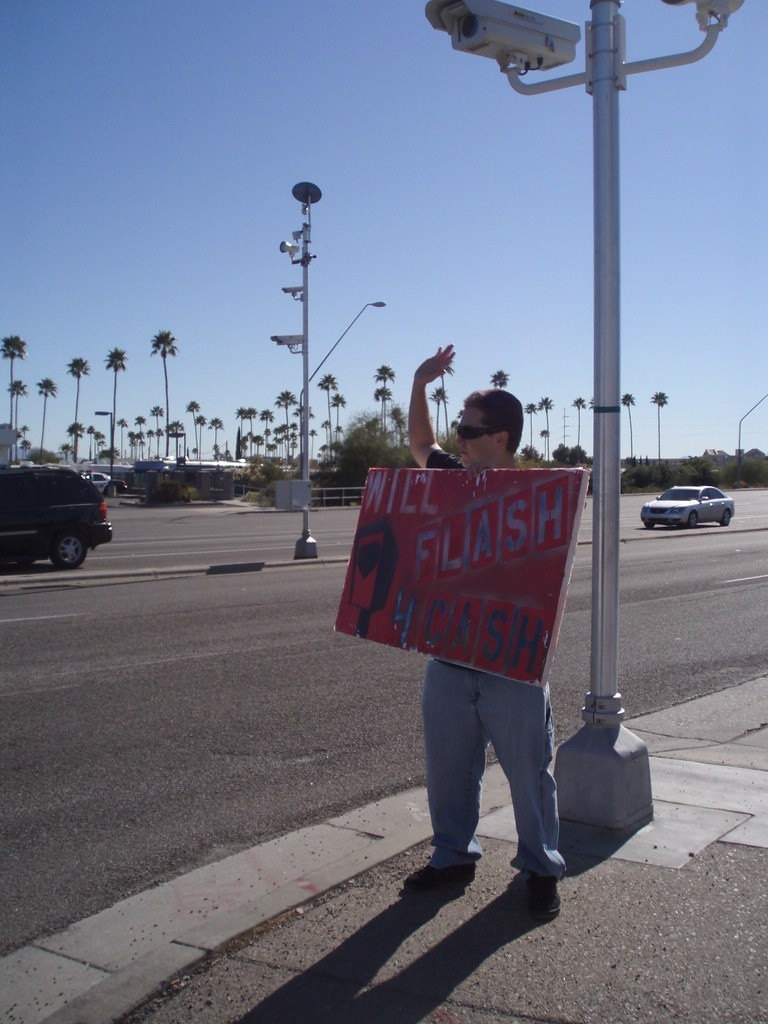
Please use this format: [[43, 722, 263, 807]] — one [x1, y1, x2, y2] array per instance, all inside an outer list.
[[456, 425, 501, 440]]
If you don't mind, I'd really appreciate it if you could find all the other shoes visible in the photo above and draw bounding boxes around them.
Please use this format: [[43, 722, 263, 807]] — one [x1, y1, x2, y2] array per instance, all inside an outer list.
[[527, 874, 561, 922], [403, 864, 477, 890]]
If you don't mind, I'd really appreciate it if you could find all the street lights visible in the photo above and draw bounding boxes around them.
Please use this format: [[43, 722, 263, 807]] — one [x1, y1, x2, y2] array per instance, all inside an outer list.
[[94, 411, 117, 498], [301, 301, 385, 479], [269, 180, 323, 557], [168, 432, 187, 458]]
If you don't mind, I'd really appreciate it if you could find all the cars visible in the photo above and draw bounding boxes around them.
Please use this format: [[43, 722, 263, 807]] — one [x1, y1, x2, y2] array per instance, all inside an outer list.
[[640, 486, 736, 530], [79, 470, 127, 495]]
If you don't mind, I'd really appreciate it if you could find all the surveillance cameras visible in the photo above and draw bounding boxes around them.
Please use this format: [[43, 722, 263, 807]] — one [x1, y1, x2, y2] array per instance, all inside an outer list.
[[423, 0, 581, 71]]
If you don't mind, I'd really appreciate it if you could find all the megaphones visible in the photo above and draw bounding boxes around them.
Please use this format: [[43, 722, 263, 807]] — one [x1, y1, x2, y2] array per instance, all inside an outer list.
[[280, 240, 299, 253]]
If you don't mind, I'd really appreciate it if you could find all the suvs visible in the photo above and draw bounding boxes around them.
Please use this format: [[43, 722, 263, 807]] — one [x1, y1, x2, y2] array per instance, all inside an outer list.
[[0, 462, 113, 570]]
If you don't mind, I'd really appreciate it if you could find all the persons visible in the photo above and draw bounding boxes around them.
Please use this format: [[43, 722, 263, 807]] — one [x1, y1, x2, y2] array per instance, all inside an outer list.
[[403, 344, 566, 921]]
